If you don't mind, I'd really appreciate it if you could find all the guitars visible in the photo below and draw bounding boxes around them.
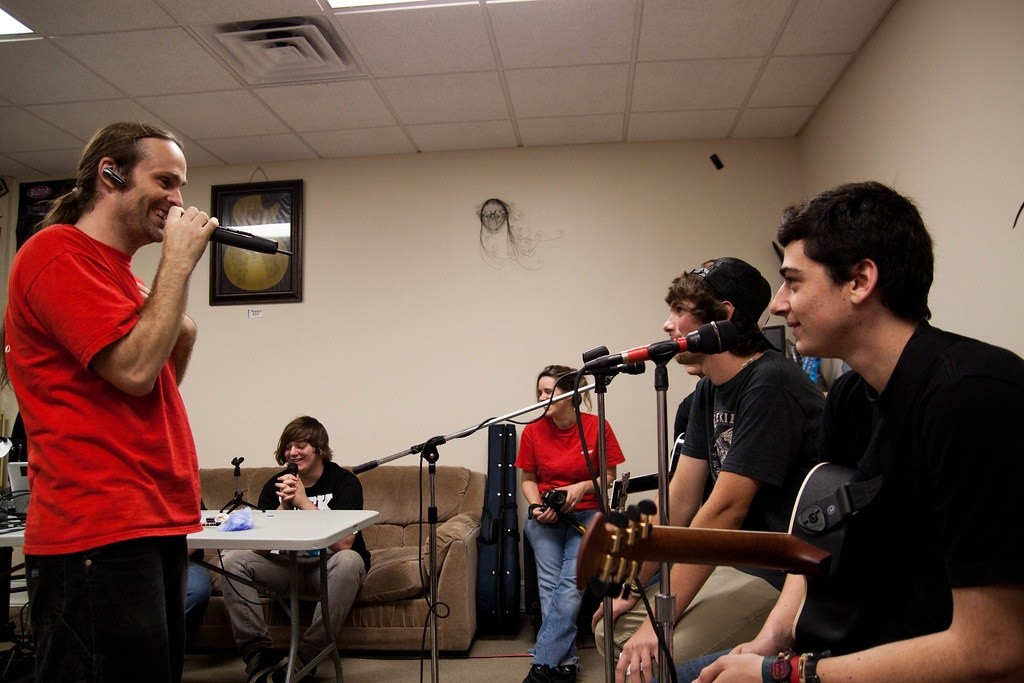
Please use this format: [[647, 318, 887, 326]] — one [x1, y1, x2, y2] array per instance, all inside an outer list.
[[576, 460, 856, 591]]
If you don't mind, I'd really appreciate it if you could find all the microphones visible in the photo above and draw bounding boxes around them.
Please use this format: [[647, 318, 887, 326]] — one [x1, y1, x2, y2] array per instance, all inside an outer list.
[[286, 462, 298, 477], [180, 212, 293, 257], [570, 320, 736, 377]]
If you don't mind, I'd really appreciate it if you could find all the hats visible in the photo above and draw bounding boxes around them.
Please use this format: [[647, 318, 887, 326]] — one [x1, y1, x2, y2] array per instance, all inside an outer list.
[[691, 255, 782, 352]]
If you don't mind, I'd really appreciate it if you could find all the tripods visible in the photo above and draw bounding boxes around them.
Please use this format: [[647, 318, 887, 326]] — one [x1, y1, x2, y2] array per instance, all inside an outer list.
[[220, 457, 266, 515]]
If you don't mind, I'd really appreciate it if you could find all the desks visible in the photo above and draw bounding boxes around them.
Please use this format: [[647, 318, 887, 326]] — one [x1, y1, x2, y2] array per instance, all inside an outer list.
[[0, 507, 381, 683]]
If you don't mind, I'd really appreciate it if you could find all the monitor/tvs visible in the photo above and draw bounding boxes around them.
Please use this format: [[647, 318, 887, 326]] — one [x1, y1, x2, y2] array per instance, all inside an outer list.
[[6, 462, 29, 514]]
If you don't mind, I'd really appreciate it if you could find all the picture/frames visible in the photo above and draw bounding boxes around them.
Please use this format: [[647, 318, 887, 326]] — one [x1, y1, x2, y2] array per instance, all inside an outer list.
[[208, 178, 304, 307]]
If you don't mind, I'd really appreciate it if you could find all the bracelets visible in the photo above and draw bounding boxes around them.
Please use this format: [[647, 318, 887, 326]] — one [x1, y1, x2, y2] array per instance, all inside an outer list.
[[798, 651, 830, 683]]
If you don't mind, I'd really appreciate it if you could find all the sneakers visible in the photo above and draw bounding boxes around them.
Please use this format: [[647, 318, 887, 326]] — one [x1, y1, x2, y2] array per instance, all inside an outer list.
[[521, 663, 578, 683]]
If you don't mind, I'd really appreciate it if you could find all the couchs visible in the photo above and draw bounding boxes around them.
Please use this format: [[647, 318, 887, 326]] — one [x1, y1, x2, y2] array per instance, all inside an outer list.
[[185, 462, 489, 659]]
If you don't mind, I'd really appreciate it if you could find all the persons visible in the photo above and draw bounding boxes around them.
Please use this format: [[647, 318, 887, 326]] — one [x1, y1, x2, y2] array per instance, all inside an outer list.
[[182, 503, 213, 651], [1, 122, 220, 683], [514, 364, 627, 683], [638, 182, 1024, 683], [591, 269, 826, 683], [221, 416, 373, 683]]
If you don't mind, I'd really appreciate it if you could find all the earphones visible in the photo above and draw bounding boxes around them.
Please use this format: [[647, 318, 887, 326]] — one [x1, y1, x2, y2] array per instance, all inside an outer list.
[[102, 168, 126, 188]]
